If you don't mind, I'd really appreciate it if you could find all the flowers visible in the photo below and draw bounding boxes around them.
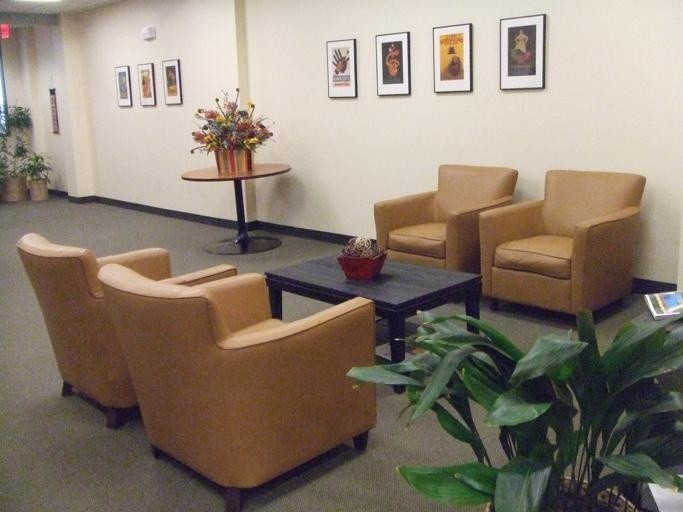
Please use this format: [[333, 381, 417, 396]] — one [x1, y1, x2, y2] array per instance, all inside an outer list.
[[186, 85, 275, 157]]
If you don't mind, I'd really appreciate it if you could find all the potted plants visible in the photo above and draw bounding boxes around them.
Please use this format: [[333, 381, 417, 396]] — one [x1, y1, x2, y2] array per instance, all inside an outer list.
[[352, 301, 683, 512], [0, 101, 32, 201], [23, 148, 54, 202]]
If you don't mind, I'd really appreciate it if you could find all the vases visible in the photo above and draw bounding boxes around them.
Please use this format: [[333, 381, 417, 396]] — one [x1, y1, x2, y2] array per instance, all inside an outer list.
[[212, 146, 253, 174]]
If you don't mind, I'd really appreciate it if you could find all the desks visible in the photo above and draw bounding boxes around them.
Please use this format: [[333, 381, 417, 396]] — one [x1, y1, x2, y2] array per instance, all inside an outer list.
[[181, 161, 291, 256], [264, 256, 482, 393]]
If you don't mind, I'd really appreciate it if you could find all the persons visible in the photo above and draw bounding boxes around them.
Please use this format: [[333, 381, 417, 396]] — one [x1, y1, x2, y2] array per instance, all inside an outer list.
[[643, 290, 683, 320]]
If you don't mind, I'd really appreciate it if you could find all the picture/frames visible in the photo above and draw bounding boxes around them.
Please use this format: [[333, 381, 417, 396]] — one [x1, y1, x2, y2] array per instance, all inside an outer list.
[[161, 59, 182, 105], [498, 13, 545, 89], [136, 63, 156, 106], [325, 38, 357, 98], [432, 22, 472, 92], [115, 65, 132, 107], [375, 31, 410, 96]]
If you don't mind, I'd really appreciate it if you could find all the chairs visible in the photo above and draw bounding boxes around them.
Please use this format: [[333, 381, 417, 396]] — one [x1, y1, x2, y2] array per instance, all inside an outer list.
[[374, 162, 515, 303], [477, 167, 645, 323], [11, 229, 237, 430], [99, 258, 377, 512]]
